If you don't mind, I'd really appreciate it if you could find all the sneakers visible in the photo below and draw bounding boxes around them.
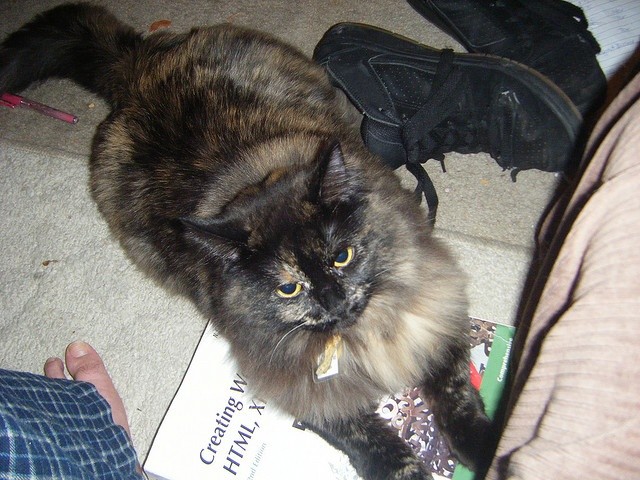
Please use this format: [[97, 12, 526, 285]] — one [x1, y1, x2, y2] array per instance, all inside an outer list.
[[311, 23, 584, 231], [407, 0, 608, 120]]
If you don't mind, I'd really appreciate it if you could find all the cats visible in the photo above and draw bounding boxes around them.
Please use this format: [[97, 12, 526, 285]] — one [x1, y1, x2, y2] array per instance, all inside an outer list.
[[0, 0, 499, 480]]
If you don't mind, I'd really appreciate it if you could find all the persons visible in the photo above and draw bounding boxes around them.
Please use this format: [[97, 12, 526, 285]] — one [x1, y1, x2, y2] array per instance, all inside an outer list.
[[0, 340, 143, 480]]
